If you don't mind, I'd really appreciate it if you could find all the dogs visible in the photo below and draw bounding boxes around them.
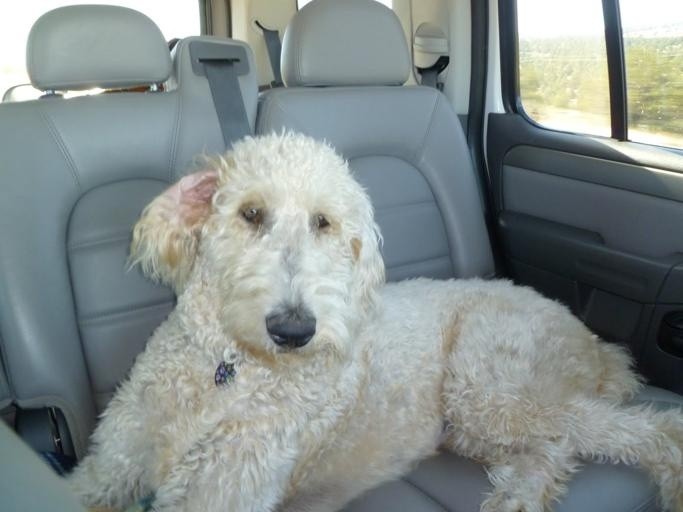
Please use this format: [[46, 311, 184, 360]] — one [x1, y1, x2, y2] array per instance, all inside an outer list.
[[61, 125, 683, 512]]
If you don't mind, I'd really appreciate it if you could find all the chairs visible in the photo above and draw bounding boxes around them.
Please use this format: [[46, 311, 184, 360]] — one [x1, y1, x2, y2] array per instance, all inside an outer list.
[[1, 0, 448, 509], [253, 0, 680, 512]]
